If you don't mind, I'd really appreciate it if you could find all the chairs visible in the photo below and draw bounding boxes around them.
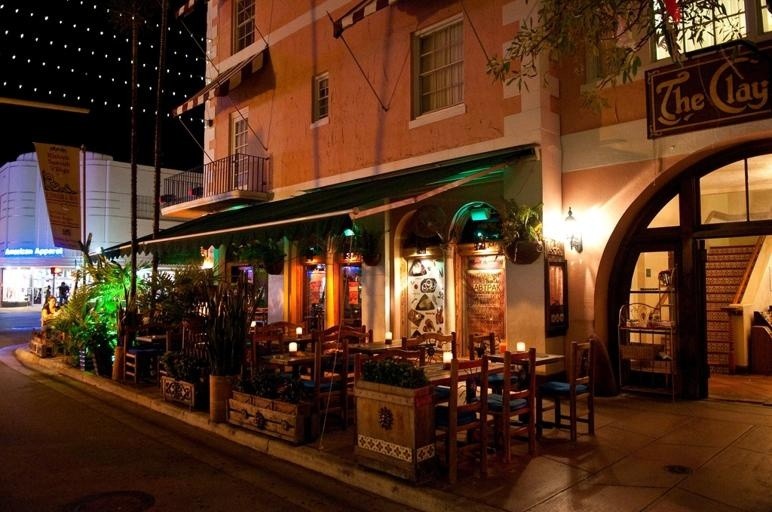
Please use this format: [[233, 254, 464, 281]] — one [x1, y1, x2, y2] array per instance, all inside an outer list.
[[123, 322, 597, 485]]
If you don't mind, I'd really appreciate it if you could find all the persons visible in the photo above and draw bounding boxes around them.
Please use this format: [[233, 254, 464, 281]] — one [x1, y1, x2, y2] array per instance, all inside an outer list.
[[47, 286, 51, 298], [35, 289, 41, 301], [58, 282, 69, 304]]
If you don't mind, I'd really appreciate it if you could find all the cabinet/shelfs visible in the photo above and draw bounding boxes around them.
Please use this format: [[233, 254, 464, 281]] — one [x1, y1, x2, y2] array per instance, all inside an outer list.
[[618, 302, 680, 400]]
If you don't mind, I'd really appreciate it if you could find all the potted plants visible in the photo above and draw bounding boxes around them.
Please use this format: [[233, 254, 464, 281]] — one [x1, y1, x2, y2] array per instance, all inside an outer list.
[[51, 256, 145, 378], [487, 195, 545, 262], [257, 240, 285, 275], [353, 228, 383, 268]]
